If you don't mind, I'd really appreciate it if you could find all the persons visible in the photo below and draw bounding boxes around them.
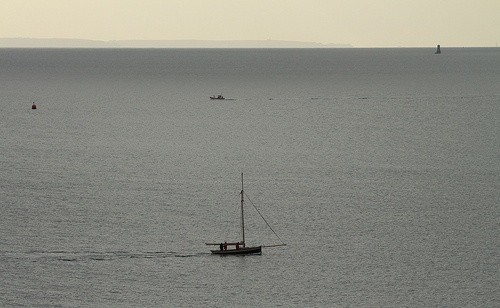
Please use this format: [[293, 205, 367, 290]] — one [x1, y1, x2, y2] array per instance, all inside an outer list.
[[32, 102, 36, 109], [224, 242, 227, 250], [220, 243, 223, 251], [236, 242, 239, 249]]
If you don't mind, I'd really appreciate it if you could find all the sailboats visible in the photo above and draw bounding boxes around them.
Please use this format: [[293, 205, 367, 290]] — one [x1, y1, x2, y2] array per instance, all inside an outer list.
[[205, 172, 286, 255]]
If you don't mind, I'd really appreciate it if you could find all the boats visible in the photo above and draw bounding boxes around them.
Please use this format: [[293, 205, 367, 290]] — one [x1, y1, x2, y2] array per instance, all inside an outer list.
[[209, 94, 225, 100]]
